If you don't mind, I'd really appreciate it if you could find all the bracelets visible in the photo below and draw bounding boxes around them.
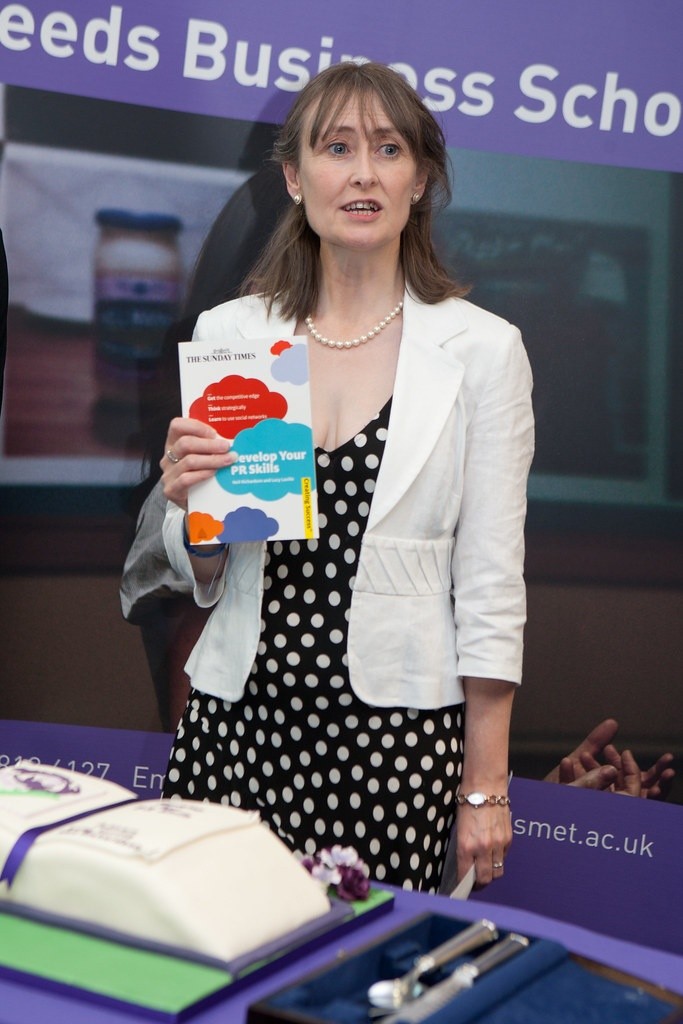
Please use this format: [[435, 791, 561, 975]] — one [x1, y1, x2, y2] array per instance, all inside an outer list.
[[455, 791, 511, 808]]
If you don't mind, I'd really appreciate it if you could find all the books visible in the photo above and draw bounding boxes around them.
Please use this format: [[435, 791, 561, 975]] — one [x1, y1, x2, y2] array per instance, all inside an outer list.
[[175, 334, 319, 547]]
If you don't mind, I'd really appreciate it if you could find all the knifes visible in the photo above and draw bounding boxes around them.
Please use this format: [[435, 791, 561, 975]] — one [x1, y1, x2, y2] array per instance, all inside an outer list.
[[383, 934, 529, 1024]]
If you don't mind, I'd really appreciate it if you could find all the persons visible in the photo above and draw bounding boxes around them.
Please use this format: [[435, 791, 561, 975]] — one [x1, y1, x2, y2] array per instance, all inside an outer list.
[[157, 62, 536, 897]]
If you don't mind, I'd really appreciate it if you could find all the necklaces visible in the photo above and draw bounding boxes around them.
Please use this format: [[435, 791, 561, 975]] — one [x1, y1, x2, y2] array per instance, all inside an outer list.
[[305, 294, 404, 350]]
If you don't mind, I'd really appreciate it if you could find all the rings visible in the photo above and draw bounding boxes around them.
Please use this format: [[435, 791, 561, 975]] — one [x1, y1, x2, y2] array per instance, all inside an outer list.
[[167, 449, 179, 465], [492, 861, 503, 869]]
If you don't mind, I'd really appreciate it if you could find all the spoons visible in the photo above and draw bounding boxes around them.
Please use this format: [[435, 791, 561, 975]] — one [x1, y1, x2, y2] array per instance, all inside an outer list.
[[367, 919, 498, 1008]]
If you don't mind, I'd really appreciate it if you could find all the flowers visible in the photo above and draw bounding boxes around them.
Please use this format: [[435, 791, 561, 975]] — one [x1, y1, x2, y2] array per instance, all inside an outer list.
[[298, 844, 375, 905]]
